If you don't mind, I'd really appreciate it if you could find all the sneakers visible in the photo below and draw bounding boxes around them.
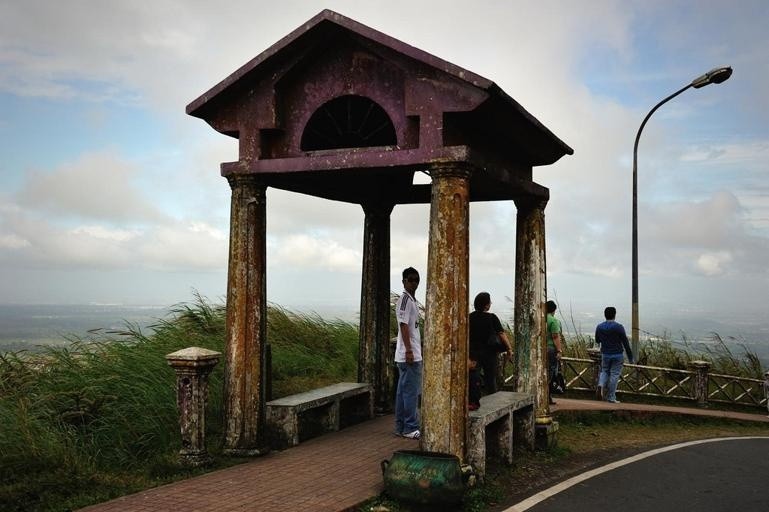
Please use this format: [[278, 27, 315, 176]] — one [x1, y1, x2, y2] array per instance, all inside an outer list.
[[609, 400, 621, 403], [596, 384, 603, 401], [402, 430, 420, 439], [392, 432, 402, 438]]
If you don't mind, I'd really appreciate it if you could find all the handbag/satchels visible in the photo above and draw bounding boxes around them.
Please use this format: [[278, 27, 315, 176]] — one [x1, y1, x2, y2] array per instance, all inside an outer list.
[[550, 358, 566, 395]]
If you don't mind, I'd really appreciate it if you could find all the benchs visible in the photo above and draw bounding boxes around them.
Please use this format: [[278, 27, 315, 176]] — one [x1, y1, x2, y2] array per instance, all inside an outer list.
[[266, 382, 374, 446], [467, 390, 536, 484]]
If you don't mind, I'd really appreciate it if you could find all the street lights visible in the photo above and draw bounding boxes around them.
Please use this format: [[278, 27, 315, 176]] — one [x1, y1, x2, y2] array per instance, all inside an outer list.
[[631, 63, 732, 370]]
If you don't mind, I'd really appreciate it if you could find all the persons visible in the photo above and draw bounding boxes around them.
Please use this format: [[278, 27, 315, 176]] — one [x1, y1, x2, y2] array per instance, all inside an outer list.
[[468, 292, 512, 394], [468, 352, 482, 411], [595, 306, 636, 403], [546, 301, 563, 405], [393, 267, 424, 440]]
